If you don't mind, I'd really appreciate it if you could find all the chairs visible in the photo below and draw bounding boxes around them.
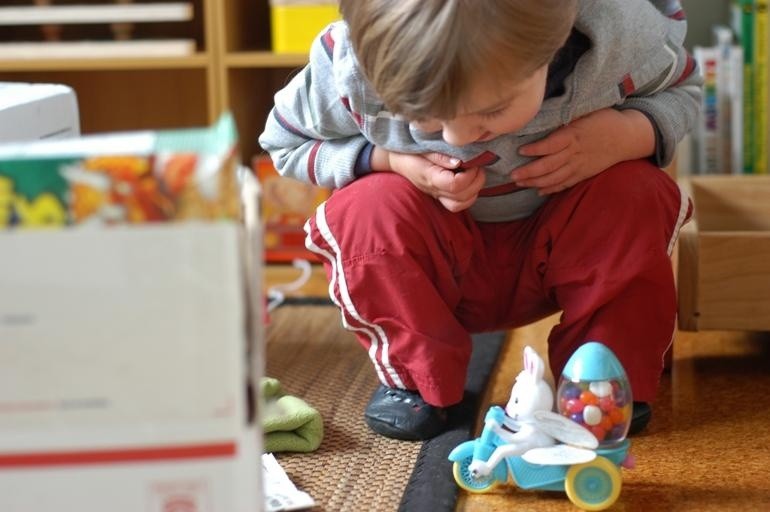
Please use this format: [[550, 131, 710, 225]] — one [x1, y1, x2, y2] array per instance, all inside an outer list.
[[627, 401, 652, 434], [364, 384, 449, 442]]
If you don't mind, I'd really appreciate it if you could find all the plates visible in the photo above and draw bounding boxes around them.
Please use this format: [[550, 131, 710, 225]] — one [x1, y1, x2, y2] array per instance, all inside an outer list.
[[0, 0, 342, 173]]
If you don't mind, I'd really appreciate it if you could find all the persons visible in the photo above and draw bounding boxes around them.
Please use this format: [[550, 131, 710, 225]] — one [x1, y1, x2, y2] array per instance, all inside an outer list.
[[256, 0, 706, 441]]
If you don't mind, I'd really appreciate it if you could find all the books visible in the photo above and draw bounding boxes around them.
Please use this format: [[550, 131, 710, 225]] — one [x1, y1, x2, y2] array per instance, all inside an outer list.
[[0, 165, 263, 512]]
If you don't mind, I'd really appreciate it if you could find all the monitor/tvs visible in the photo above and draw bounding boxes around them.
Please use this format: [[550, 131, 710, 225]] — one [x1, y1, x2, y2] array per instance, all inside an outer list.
[[694, 0, 770, 175]]
